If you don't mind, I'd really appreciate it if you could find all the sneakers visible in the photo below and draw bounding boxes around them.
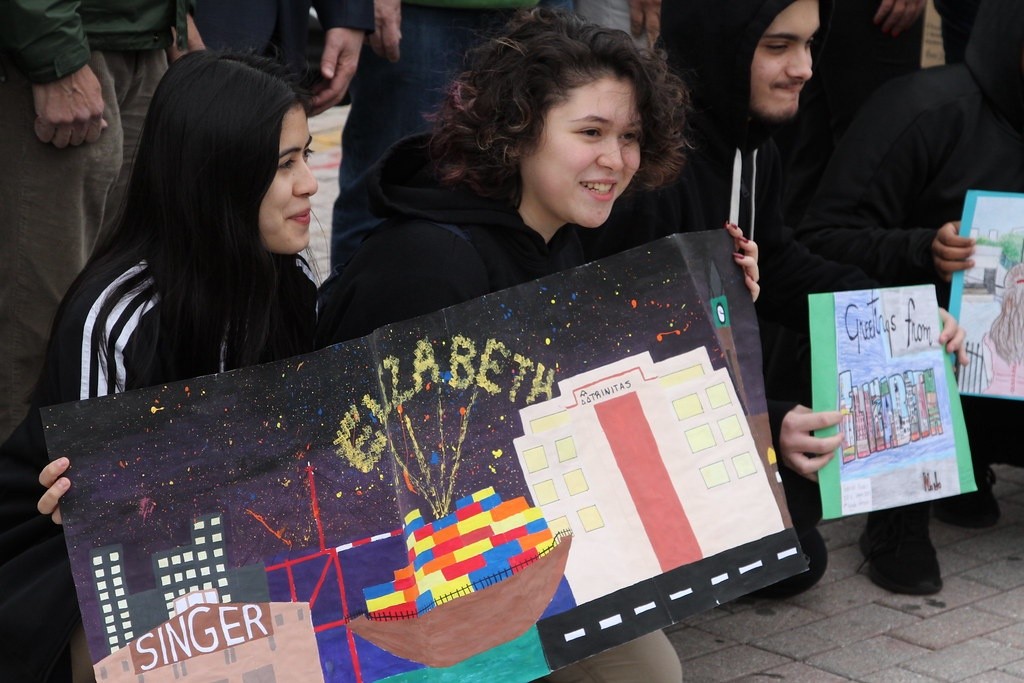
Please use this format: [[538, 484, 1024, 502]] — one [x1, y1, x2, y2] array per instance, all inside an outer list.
[[856, 507, 942, 593]]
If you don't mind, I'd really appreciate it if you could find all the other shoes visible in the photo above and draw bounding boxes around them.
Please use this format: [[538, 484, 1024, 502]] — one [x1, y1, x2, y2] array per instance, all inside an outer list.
[[932, 458, 1000, 529]]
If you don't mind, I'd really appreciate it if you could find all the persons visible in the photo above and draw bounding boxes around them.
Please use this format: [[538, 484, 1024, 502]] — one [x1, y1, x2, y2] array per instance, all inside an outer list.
[[315, 4, 760, 682], [1, 46, 324, 683], [0, 0, 1023, 592]]
